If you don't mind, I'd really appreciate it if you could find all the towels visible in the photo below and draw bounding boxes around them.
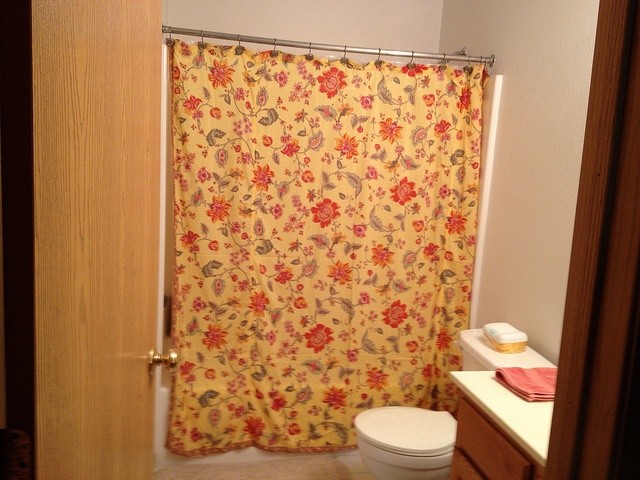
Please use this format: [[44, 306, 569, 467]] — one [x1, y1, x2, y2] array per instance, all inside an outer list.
[[496, 367, 559, 402]]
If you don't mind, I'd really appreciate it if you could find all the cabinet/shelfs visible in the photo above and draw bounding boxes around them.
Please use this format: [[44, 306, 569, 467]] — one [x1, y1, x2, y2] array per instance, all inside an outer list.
[[450, 394, 532, 479]]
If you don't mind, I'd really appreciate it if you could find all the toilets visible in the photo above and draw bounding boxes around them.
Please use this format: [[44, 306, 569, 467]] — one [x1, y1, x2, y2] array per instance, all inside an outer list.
[[354, 328, 559, 480]]
